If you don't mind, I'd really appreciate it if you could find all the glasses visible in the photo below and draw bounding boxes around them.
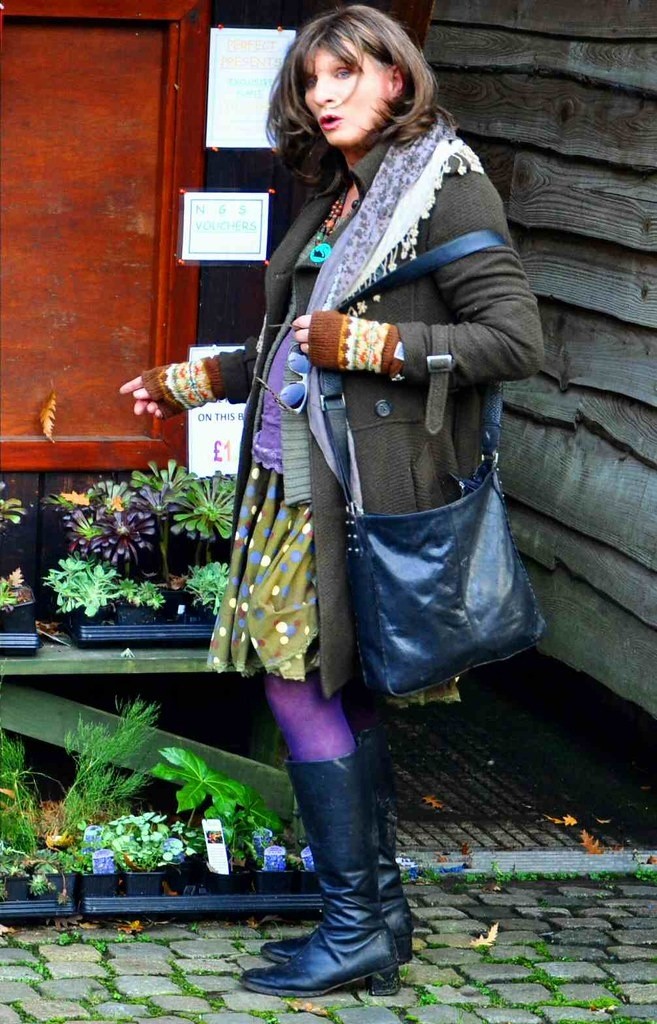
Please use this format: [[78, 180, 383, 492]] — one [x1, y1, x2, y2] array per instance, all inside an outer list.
[[256, 323, 313, 415]]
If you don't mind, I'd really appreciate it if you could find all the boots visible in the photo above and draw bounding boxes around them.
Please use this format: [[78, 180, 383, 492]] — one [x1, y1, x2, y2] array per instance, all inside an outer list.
[[259, 727, 415, 963], [239, 745, 401, 996]]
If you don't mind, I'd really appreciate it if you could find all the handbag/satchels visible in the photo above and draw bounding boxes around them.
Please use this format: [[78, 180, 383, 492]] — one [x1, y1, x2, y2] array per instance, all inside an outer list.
[[342, 476, 546, 698]]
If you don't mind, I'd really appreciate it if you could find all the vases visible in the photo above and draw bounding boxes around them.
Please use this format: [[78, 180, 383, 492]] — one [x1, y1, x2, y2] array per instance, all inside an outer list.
[[207, 869, 245, 892], [114, 588, 202, 624], [298, 870, 320, 894], [0, 586, 38, 633], [252, 868, 293, 893]]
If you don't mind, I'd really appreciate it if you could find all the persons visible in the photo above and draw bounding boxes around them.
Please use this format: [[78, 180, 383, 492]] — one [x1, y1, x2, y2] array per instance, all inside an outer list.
[[120, 6, 544, 998]]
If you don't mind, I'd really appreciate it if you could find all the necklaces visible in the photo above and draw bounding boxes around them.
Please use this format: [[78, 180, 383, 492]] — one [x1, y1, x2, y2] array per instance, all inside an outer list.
[[315, 185, 358, 247]]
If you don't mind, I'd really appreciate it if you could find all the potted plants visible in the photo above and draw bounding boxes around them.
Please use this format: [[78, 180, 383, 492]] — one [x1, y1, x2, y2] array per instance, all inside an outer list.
[[162, 821, 204, 894], [110, 813, 168, 896], [32, 850, 75, 899], [2, 867, 37, 901], [75, 841, 120, 898]]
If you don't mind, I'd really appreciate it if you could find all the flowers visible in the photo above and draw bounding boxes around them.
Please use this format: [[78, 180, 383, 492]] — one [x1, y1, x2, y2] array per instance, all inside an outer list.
[[0, 567, 25, 611], [44, 465, 228, 615]]
